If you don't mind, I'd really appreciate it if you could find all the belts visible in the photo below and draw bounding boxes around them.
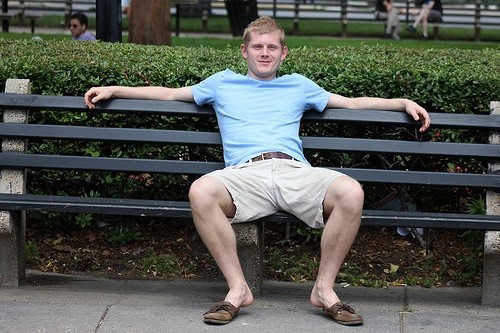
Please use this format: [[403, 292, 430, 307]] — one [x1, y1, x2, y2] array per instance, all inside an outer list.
[[247, 152, 298, 162]]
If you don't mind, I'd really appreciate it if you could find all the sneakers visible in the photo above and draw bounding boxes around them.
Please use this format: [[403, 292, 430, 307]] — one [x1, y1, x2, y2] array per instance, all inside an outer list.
[[323, 300, 365, 325], [203, 300, 240, 324]]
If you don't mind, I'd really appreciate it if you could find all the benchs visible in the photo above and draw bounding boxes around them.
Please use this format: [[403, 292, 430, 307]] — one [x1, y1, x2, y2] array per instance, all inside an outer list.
[[0, 0, 500, 43], [0, 78, 500, 309]]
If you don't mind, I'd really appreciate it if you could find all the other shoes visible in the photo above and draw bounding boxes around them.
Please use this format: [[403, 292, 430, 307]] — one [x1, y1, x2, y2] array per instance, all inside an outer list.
[[404, 25, 417, 33], [418, 32, 432, 40]]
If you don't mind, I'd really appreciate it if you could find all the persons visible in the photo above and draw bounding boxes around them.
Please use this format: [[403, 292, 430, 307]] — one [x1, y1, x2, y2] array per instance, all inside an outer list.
[[373, 0, 400, 40], [69, 12, 96, 41], [84, 16, 431, 326], [405, 0, 442, 39]]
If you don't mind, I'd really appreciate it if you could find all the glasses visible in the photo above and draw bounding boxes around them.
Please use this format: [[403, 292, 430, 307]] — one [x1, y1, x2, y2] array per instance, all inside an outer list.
[[69, 24, 78, 28]]
